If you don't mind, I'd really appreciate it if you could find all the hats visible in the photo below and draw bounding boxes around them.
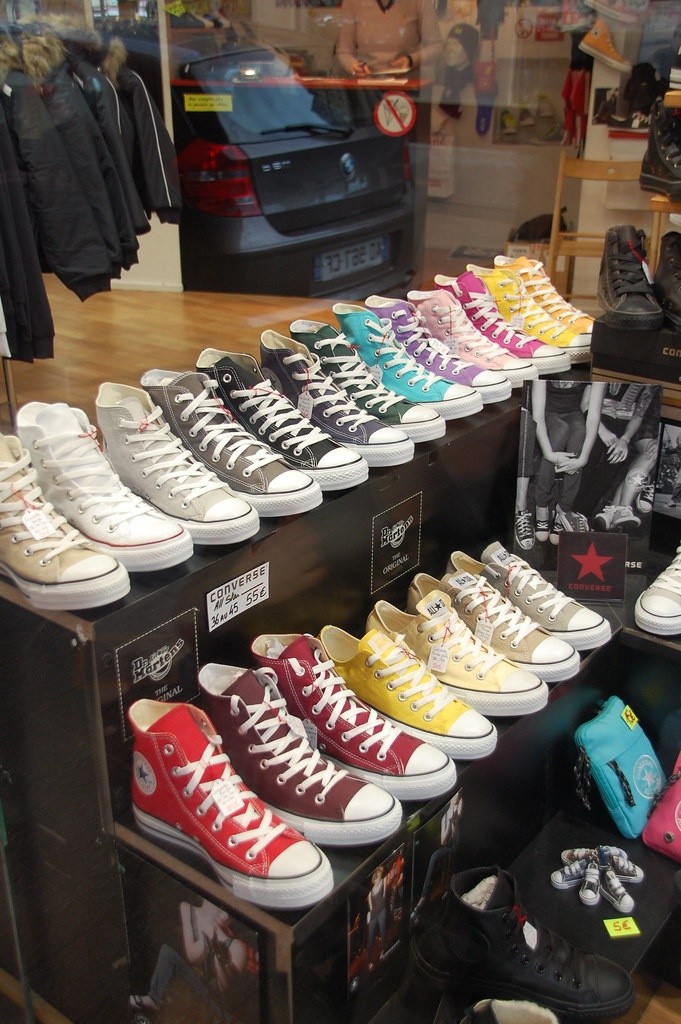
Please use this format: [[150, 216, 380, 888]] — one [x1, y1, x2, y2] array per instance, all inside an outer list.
[[623, 62, 658, 102], [448, 23, 479, 62]]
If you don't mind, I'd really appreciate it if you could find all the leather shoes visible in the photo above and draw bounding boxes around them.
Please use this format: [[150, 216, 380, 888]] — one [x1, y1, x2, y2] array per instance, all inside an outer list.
[[408, 864, 634, 1024]]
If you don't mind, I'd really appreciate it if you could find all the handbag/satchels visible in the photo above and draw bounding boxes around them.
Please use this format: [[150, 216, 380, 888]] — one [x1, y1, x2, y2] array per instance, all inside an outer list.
[[573, 695, 681, 859], [517, 206, 568, 241]]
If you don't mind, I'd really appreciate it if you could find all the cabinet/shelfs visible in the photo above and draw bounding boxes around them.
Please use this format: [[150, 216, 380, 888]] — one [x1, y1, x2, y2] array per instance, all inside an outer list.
[[0, 356, 681, 1024]]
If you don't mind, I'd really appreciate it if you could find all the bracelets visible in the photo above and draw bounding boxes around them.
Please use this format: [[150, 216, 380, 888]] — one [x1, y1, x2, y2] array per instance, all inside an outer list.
[[621, 436, 628, 445], [405, 55, 413, 68]]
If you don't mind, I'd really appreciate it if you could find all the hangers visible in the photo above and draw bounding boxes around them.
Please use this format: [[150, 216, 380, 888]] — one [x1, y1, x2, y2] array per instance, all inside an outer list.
[[0, 22, 133, 91]]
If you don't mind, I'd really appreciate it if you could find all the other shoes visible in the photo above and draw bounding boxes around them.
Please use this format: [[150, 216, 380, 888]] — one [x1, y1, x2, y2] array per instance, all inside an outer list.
[[663, 501, 677, 509]]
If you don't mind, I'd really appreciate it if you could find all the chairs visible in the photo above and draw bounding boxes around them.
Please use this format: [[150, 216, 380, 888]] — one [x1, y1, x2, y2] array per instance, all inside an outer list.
[[547, 152, 666, 318]]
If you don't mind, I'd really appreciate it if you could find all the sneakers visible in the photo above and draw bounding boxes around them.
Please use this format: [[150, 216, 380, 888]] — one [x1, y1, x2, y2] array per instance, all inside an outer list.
[[190, 541, 616, 849], [635, 475, 654, 513], [552, 846, 645, 912], [171, 11, 230, 30], [560, 0, 638, 73], [607, 108, 652, 133], [634, 546, 681, 638], [500, 95, 566, 145], [597, 225, 681, 333], [333, 254, 597, 421], [0, 318, 446, 611], [125, 699, 334, 911], [514, 504, 592, 551], [594, 506, 641, 533], [638, 97, 681, 203]]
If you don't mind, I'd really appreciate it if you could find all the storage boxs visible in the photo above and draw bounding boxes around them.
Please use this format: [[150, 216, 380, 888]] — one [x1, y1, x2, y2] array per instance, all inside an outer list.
[[503, 239, 570, 302], [589, 317, 681, 403]]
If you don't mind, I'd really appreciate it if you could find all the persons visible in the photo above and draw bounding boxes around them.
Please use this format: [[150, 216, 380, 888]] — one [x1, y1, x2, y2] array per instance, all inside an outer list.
[[410, 787, 463, 920], [648, 23, 681, 82], [128, 897, 248, 1024], [514, 380, 681, 550], [336, 0, 444, 78], [443, 24, 480, 87], [365, 865, 387, 966]]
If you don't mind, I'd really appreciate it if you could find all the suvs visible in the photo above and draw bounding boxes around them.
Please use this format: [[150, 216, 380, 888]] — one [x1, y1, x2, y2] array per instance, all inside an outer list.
[[0, 16, 418, 298]]
